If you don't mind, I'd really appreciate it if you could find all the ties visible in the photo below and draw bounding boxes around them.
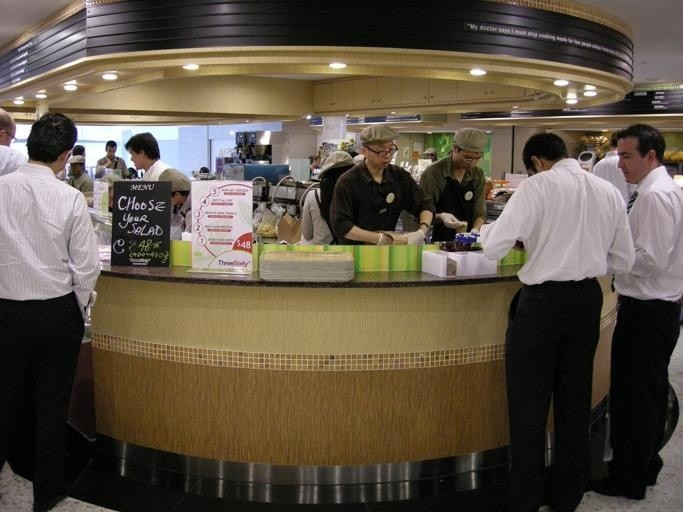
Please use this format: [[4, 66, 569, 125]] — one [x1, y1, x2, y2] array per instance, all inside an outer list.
[[611, 191, 638, 293]]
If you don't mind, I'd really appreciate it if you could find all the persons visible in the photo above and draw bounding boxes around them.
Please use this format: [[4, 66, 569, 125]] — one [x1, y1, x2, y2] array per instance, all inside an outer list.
[[0, 107, 29, 177], [300, 150, 355, 245], [309, 155, 321, 177], [590, 123, 683, 500], [591, 128, 639, 204], [348, 151, 366, 163], [417, 127, 487, 242], [199, 166, 209, 180], [423, 147, 438, 163], [55, 132, 191, 233], [329, 124, 436, 245], [0, 112, 101, 512], [479, 132, 636, 512]]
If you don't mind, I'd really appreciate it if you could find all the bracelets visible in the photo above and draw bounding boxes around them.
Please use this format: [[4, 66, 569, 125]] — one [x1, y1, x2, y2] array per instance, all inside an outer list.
[[375, 232, 384, 245], [419, 220, 429, 231]]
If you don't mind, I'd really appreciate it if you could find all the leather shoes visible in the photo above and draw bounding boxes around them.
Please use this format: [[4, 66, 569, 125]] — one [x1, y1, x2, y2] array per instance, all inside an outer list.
[[596, 460, 659, 485], [33, 487, 70, 512], [589, 471, 646, 500]]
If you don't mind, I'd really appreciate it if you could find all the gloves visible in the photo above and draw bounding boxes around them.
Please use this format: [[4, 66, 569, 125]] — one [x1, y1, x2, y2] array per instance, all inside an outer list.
[[401, 228, 425, 246], [376, 233, 392, 245], [470, 228, 479, 233], [441, 212, 463, 229]]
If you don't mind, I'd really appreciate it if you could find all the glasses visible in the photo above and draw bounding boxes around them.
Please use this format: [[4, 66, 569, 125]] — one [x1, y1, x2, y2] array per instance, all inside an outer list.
[[457, 148, 484, 161], [362, 144, 398, 156], [0, 127, 17, 143]]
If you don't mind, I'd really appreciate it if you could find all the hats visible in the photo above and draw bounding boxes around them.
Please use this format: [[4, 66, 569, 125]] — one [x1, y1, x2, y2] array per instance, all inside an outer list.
[[423, 148, 437, 154], [158, 169, 191, 192], [318, 150, 355, 179], [67, 155, 85, 164], [454, 127, 488, 153], [360, 124, 400, 142]]
